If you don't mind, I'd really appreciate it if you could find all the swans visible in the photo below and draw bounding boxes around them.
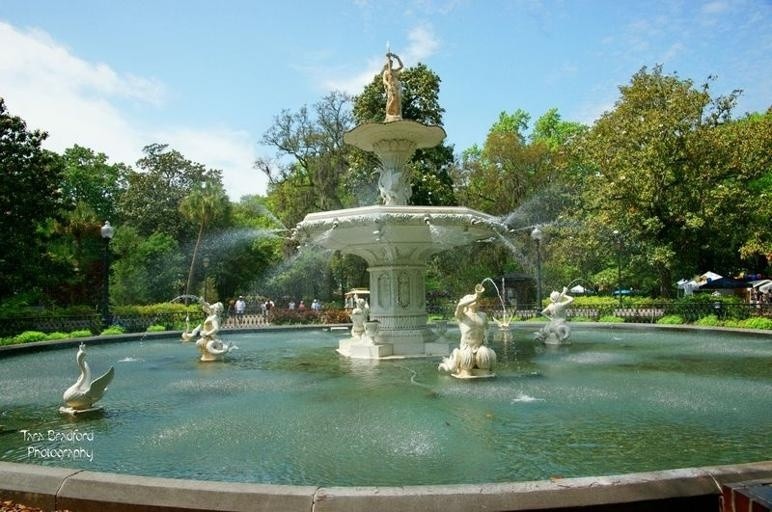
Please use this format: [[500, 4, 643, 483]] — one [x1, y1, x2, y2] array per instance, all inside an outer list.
[[493, 314, 514, 327], [63, 342, 115, 409], [182, 317, 201, 340]]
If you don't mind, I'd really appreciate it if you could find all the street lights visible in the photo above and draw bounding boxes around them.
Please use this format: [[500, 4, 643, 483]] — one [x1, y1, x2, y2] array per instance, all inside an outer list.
[[100, 219, 117, 330], [202, 254, 211, 319], [531, 224, 546, 317], [613, 230, 624, 309]]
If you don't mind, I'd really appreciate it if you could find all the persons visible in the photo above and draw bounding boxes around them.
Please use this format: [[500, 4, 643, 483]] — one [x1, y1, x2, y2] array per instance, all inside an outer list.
[[227, 300, 236, 326], [316, 299, 321, 310], [289, 300, 295, 309], [299, 300, 305, 309], [266, 299, 271, 315], [311, 299, 316, 309], [455, 294, 496, 379], [383, 52, 405, 122], [185, 296, 232, 354], [540, 291, 576, 341], [261, 301, 266, 315], [234, 296, 246, 324], [265, 298, 275, 306], [350, 292, 369, 337], [711, 288, 721, 309]]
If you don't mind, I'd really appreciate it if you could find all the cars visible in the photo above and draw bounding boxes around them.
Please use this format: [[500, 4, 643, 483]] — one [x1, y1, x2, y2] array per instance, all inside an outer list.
[[614, 288, 643, 296]]
